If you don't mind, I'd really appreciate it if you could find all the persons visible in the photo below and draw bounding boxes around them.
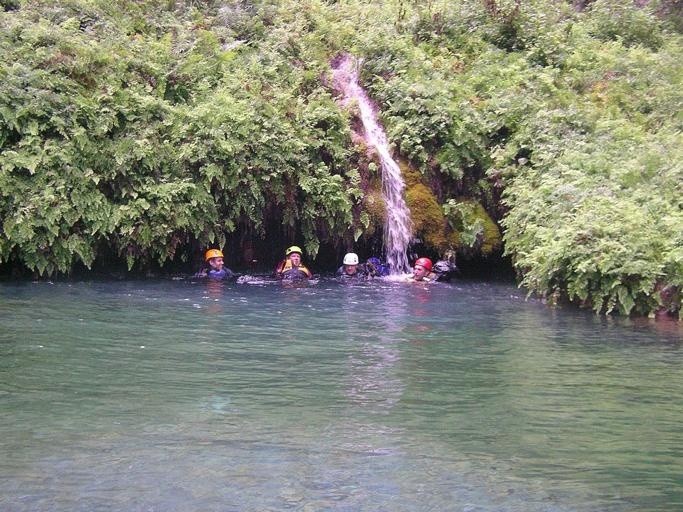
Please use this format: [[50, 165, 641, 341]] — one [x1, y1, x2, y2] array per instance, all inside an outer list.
[[274, 245, 312, 279], [400, 257, 438, 286], [204, 248, 234, 277], [336, 252, 374, 284]]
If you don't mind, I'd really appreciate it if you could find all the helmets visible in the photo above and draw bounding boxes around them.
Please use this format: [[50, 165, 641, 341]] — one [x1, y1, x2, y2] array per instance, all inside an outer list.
[[205, 248, 224, 262], [342, 253, 359, 265], [286, 246, 302, 255], [416, 257, 433, 271]]
